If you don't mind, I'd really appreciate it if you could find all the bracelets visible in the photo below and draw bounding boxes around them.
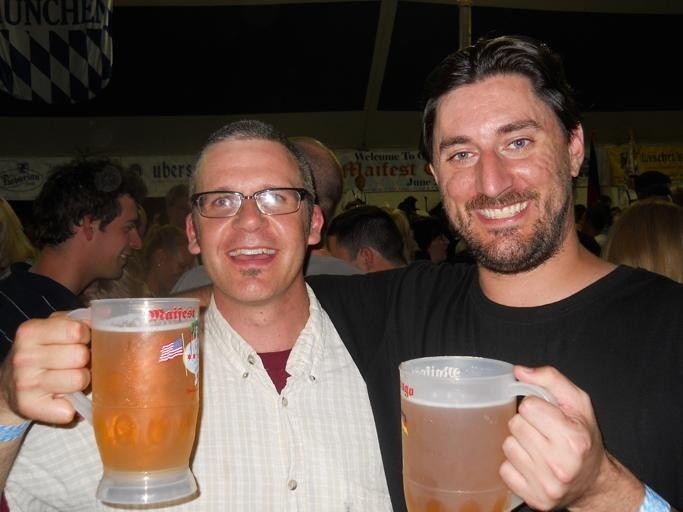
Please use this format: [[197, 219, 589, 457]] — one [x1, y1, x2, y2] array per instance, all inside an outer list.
[[638, 485, 671, 512], [0, 420, 33, 441]]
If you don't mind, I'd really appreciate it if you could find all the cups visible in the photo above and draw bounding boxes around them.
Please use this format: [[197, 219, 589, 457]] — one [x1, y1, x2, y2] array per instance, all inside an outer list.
[[60, 292, 207, 510], [394, 353, 558, 512]]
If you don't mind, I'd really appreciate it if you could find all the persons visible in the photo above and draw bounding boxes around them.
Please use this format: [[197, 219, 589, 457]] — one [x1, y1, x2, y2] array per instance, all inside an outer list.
[[6, 118, 394, 510], [0, 138, 682, 354], [9, 36, 682, 512]]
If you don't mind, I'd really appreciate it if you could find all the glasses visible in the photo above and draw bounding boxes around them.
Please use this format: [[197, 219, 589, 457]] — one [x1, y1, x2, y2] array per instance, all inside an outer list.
[[186, 186, 309, 220]]
